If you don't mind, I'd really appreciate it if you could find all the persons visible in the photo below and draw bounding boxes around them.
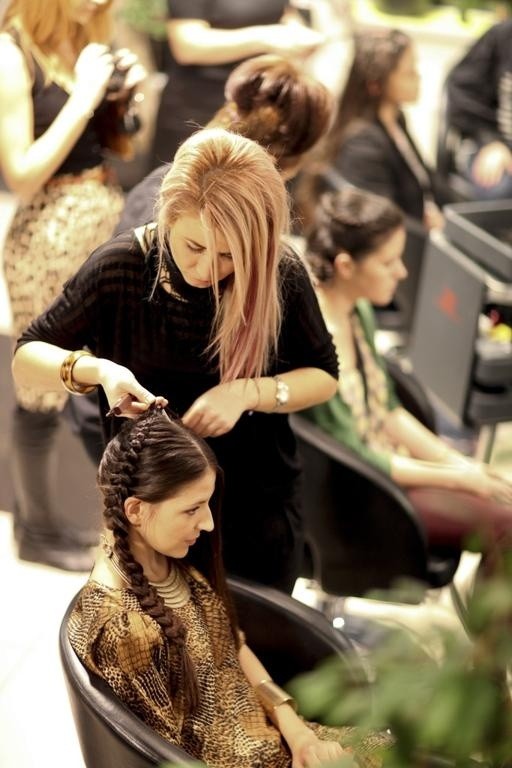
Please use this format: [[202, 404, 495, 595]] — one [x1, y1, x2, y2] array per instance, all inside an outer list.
[[68, 399, 393, 768], [2, 1, 147, 341], [293, 183, 510, 702], [327, 26, 442, 314], [436, 18, 511, 329], [10, 126, 341, 596], [115, 55, 336, 239], [153, 1, 325, 176]]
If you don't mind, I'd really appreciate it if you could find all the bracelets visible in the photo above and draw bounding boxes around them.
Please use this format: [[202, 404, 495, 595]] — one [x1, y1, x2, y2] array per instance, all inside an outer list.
[[255, 679, 297, 718], [59, 349, 97, 396], [250, 379, 261, 413]]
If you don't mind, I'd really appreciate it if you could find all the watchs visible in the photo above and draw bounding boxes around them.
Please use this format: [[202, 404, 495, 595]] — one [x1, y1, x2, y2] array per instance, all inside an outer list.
[[268, 374, 290, 414]]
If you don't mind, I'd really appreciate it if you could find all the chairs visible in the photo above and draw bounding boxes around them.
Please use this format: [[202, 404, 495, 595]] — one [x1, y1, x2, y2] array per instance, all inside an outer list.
[[288, 353, 460, 604], [59, 568, 373, 768], [309, 165, 468, 336]]
